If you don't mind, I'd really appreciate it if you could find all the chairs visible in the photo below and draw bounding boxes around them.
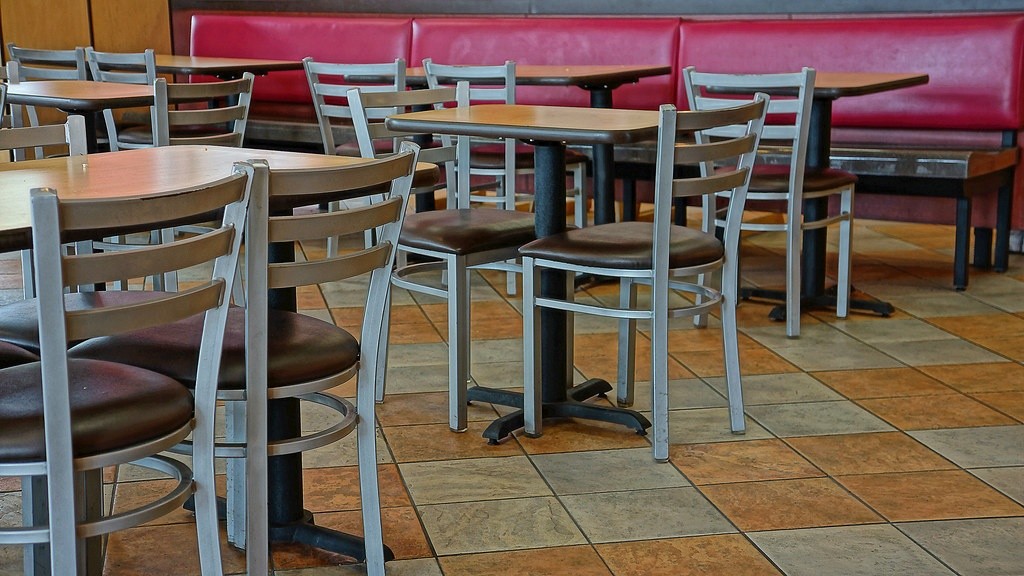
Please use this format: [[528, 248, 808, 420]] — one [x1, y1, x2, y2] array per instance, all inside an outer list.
[[0, 45, 853, 575]]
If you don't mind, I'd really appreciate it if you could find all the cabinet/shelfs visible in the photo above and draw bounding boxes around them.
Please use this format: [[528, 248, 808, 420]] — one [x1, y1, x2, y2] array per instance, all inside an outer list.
[[0, 1, 171, 161]]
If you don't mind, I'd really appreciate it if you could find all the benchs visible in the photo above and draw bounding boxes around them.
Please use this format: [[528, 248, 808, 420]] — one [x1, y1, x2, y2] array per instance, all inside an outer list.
[[172, 12, 414, 146], [408, 15, 681, 201], [676, 13, 1023, 290]]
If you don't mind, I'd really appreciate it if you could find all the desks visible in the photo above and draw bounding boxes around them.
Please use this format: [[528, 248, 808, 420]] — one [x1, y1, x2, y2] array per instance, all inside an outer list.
[[735, 71, 926, 317]]
